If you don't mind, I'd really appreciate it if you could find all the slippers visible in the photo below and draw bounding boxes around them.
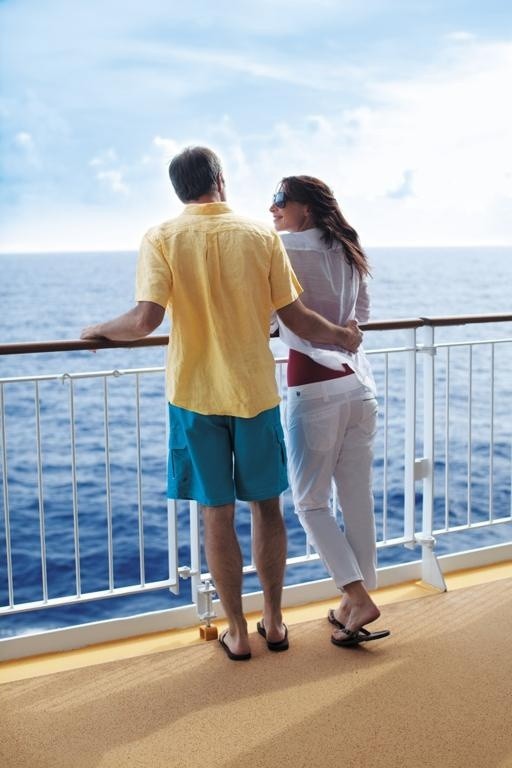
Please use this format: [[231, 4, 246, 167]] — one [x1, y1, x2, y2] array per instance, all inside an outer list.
[[327, 608, 346, 629], [257, 618, 290, 652], [330, 626, 390, 647], [218, 630, 251, 661]]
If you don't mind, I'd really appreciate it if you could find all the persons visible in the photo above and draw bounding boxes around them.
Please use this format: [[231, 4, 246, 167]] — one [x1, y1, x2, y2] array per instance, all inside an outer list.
[[269, 175, 390, 645], [81, 145, 363, 661]]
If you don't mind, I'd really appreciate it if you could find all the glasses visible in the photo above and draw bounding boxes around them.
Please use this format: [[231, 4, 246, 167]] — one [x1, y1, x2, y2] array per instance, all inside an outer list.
[[273, 191, 299, 209]]
